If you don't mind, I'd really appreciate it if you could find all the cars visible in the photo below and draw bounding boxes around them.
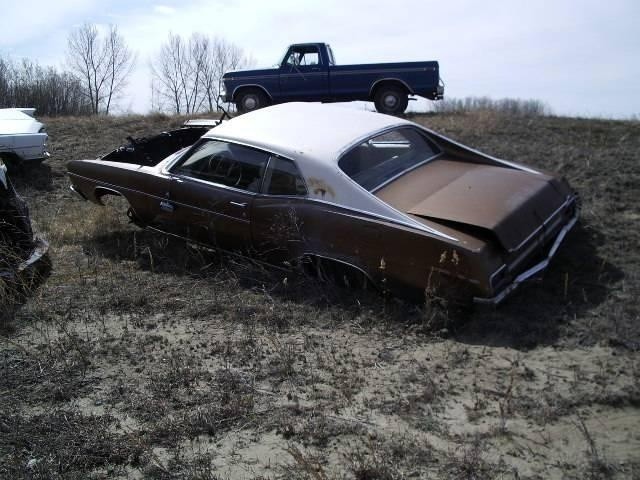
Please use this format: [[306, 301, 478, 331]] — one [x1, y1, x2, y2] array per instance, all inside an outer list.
[[1, 103, 52, 173], [62, 98, 583, 323]]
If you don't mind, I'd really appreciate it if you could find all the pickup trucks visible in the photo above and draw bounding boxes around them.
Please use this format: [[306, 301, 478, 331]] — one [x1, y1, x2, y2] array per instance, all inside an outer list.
[[217, 42, 445, 111]]
